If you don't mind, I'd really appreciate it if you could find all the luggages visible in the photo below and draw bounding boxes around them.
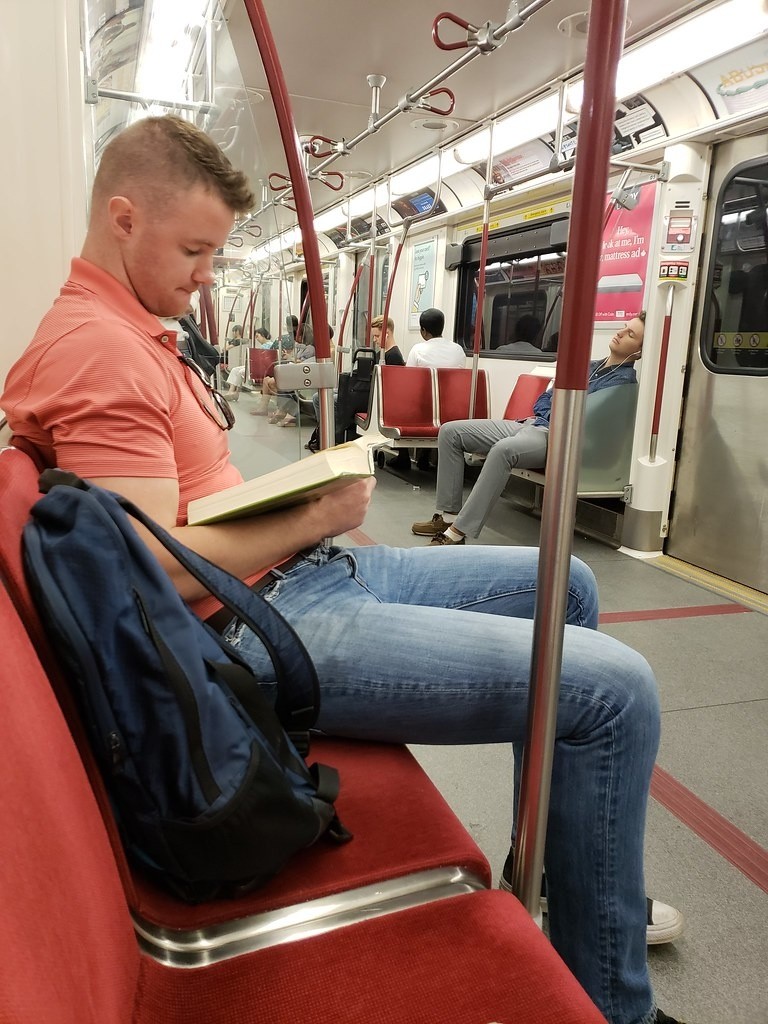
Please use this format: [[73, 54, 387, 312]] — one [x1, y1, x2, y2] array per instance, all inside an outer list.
[[334, 348, 376, 413]]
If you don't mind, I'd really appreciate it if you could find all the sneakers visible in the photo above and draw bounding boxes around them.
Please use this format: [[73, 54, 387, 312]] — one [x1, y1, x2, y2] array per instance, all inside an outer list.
[[426, 530, 465, 546], [411, 513, 453, 536], [498, 844, 687, 946]]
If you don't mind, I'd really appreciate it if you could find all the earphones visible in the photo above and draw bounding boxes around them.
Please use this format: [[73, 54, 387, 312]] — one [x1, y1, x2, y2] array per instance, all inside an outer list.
[[634, 351, 641, 355]]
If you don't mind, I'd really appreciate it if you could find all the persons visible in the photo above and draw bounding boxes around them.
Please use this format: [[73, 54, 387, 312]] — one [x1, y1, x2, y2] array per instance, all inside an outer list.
[[220, 316, 335, 427], [304, 316, 405, 450], [412, 310, 646, 547], [497, 313, 545, 351], [386, 307, 466, 472], [0, 114, 686, 1024]]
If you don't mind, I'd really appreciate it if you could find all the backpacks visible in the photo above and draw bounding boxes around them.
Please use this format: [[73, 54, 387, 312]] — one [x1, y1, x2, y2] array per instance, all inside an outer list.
[[19, 463, 354, 903]]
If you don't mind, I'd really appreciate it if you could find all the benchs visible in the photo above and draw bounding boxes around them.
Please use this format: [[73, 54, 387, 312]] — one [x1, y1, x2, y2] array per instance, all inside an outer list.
[[0, 441, 611, 1024], [228, 344, 638, 500]]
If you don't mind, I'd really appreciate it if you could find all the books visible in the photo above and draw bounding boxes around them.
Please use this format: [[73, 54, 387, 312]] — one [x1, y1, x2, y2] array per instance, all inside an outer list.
[[188, 432, 394, 526]]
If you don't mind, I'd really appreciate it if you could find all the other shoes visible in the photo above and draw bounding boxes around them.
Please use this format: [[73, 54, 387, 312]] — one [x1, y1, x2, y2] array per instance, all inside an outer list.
[[249, 410, 268, 416], [223, 393, 239, 402], [277, 417, 297, 426], [265, 414, 286, 424], [304, 442, 318, 449]]
